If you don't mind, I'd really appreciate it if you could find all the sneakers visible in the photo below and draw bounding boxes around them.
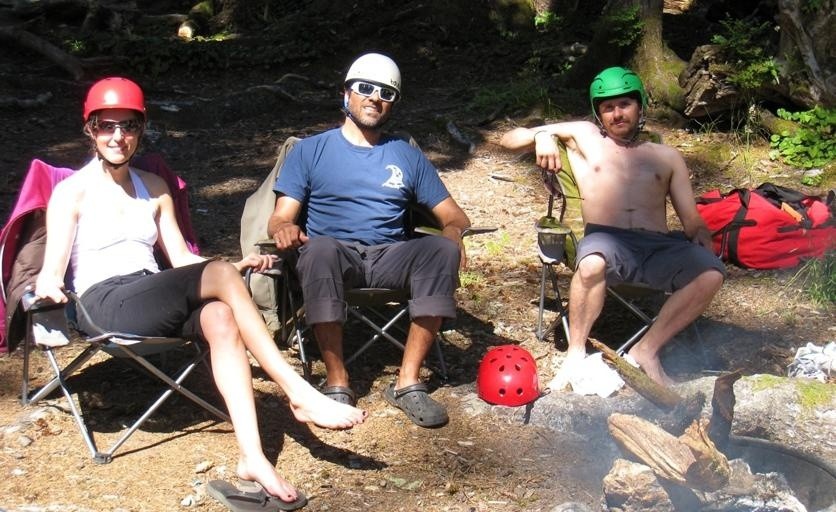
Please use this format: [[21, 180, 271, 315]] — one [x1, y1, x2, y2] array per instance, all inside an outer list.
[[786, 357, 828, 386], [794, 342, 836, 372]]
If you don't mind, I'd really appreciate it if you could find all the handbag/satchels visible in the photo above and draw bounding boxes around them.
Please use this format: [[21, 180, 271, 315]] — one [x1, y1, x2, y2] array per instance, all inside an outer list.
[[694, 183, 836, 270]]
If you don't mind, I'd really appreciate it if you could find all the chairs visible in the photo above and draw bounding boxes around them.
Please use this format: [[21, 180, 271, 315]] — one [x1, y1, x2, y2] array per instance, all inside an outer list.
[[258, 136, 453, 384], [535, 127, 713, 368], [20, 156, 257, 461]]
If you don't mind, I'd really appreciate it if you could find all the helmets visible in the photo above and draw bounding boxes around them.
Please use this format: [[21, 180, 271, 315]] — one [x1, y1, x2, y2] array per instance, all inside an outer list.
[[479, 346, 541, 406], [84, 77, 146, 122], [345, 54, 401, 94], [589, 67, 647, 119]]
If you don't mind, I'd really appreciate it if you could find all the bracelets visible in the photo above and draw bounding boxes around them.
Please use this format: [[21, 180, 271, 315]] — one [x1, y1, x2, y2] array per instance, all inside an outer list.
[[533, 129, 547, 141]]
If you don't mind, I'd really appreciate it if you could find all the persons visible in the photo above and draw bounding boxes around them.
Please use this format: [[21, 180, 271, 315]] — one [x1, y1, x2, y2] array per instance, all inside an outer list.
[[267, 53, 472, 428], [499, 65, 727, 392], [38, 76, 370, 502]]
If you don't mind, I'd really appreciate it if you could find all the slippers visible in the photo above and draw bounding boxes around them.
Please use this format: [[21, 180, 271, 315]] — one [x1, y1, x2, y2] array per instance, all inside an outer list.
[[321, 386, 356, 408], [383, 384, 449, 427], [209, 480, 279, 512], [237, 478, 307, 511]]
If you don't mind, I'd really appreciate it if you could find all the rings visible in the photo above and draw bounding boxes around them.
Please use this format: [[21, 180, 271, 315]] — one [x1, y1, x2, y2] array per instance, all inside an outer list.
[[274, 240, 280, 243]]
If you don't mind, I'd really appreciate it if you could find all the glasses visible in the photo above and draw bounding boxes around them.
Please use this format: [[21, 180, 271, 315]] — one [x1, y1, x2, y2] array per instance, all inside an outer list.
[[350, 81, 396, 103], [93, 119, 141, 133]]
